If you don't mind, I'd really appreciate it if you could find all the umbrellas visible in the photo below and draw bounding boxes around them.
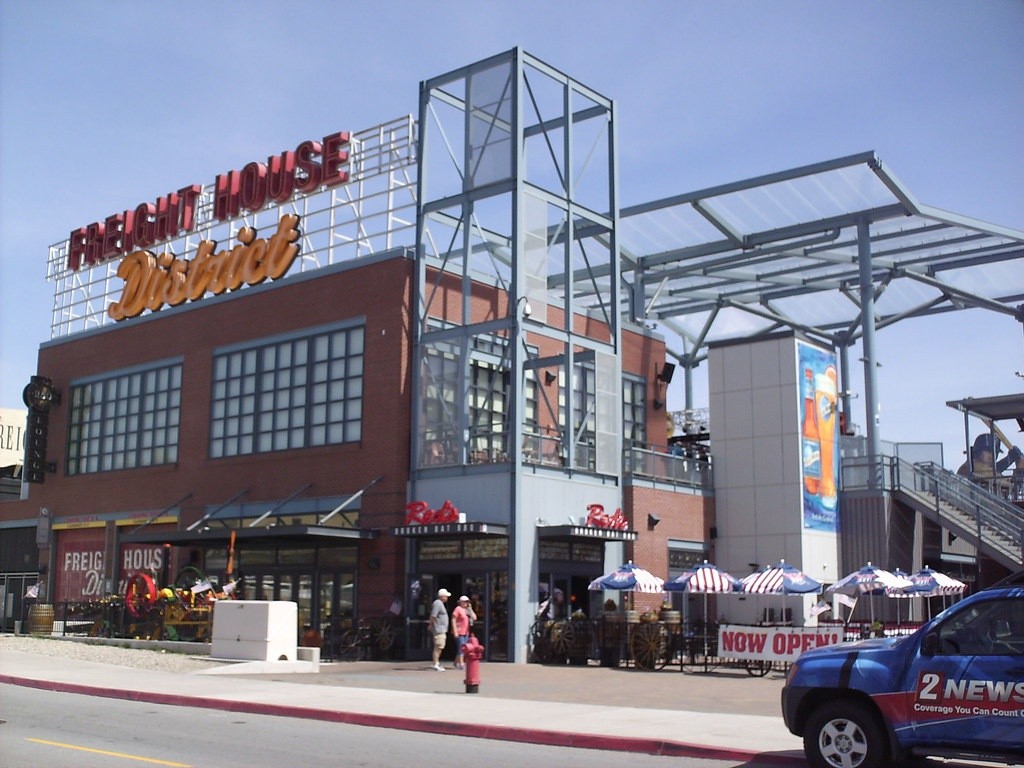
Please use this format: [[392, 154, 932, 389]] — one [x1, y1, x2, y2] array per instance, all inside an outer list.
[[587, 558, 665, 641], [663, 559, 741, 656], [735, 558, 823, 627], [826, 561, 965, 626]]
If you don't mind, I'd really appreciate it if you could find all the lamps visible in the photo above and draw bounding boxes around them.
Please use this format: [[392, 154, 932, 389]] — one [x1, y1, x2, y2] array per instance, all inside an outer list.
[[544, 370, 556, 382], [650, 514, 660, 528], [653, 399, 663, 410]]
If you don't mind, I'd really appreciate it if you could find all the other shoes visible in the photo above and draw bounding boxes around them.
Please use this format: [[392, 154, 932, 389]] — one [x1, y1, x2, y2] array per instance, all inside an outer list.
[[431, 664, 445, 672], [459, 664, 464, 669], [454, 663, 459, 669]]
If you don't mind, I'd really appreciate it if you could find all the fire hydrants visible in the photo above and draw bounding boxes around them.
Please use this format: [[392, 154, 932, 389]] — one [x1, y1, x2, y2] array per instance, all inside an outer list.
[[460, 632, 484, 693]]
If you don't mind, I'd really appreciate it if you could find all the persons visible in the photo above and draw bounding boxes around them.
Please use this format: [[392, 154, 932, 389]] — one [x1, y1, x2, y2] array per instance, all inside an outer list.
[[957, 434, 1022, 478], [427, 589, 452, 672], [451, 595, 477, 669]]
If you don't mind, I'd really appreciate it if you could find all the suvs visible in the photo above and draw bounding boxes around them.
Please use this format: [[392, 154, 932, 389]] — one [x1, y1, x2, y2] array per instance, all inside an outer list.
[[780, 569, 1024, 768]]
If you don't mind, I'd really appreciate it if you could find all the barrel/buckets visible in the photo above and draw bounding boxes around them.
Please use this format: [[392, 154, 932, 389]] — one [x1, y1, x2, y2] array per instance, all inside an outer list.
[[28, 604, 55, 635]]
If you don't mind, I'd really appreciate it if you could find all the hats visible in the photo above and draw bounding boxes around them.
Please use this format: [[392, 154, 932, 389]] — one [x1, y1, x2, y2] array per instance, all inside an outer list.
[[460, 596, 469, 601], [438, 588, 451, 596]]
